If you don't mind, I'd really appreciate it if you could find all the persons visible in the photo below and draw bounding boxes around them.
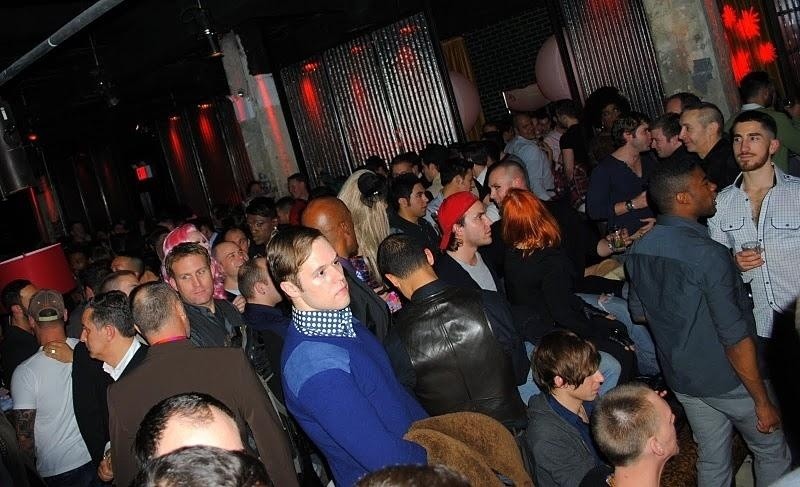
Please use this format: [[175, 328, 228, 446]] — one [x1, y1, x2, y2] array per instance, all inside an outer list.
[[0, 69, 800, 486]]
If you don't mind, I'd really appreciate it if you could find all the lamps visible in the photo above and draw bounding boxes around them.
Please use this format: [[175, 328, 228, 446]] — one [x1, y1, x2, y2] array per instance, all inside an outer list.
[[226, 90, 256, 125]]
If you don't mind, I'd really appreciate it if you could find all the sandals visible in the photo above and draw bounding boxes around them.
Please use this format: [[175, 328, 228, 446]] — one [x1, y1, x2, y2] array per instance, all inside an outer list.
[[650, 372, 670, 399]]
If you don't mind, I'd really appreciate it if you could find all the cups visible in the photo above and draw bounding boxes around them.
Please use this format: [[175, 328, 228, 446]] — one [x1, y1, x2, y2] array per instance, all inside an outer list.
[[783, 97, 794, 107], [606, 223, 628, 251], [741, 241, 762, 256]]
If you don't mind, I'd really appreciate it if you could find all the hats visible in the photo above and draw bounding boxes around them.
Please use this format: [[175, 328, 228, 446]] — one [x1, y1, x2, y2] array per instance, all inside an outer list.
[[438, 191, 479, 250], [29, 288, 65, 322]]
